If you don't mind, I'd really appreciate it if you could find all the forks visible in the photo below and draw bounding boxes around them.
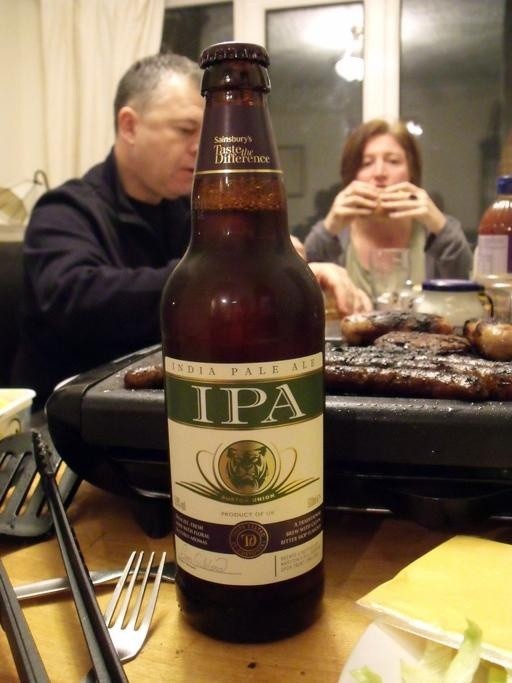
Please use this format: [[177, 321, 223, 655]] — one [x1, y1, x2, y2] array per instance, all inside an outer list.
[[89, 549, 166, 683]]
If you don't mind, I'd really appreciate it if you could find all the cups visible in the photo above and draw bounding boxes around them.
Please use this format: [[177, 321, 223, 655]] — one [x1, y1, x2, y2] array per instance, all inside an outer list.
[[369, 248, 409, 309], [484, 272, 512, 324]]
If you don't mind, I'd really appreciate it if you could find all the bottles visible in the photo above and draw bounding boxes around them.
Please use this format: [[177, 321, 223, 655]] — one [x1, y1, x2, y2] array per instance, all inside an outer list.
[[162, 41, 325, 644], [476, 175, 512, 285], [416, 278, 482, 327]]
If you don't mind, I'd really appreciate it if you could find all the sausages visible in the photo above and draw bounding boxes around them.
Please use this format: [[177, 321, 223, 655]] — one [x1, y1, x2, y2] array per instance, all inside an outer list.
[[125, 309, 511, 402]]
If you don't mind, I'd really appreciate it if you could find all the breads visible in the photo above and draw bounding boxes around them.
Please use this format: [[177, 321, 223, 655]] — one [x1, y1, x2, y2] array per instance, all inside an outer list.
[[362, 188, 398, 222]]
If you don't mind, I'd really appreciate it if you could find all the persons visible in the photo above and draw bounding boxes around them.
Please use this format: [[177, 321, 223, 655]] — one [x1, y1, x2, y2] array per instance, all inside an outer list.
[[297, 116, 476, 316], [1, 50, 377, 413]]
